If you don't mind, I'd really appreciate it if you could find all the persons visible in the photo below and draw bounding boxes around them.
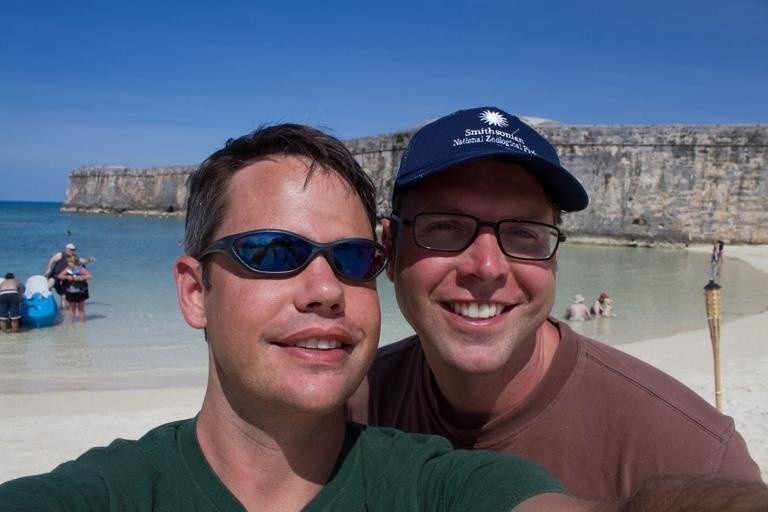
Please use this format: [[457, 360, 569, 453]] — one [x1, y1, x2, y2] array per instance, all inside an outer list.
[[565, 293, 590, 322], [0, 113, 581, 511], [42, 243, 97, 316], [590, 291, 610, 317], [711, 238, 718, 262], [601, 297, 617, 317], [346, 102, 764, 512], [717, 239, 726, 263], [57, 255, 93, 327], [0, 272, 23, 336]]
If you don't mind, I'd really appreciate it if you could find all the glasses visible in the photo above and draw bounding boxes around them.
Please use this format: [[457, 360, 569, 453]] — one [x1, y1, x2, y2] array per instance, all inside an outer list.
[[195, 230, 390, 283], [392, 212, 566, 260]]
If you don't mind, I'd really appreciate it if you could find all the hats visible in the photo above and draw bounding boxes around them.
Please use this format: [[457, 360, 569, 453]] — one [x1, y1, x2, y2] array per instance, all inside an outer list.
[[396, 108, 588, 212]]
